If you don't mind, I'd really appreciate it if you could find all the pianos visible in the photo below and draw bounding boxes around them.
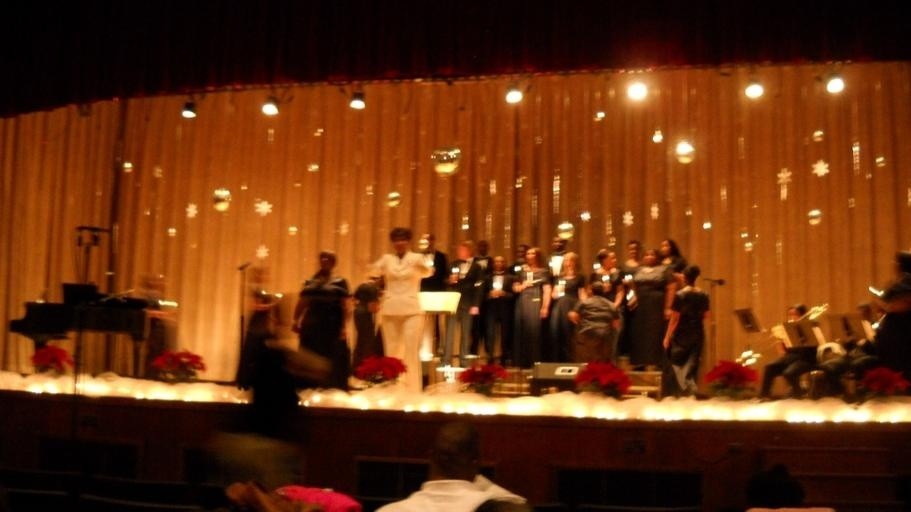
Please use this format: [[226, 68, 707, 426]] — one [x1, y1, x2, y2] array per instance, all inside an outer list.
[[10, 284, 178, 381]]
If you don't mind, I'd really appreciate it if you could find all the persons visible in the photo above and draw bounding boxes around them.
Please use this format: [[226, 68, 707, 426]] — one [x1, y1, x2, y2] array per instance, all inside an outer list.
[[227, 419, 527, 512], [763, 252, 910, 400], [254, 227, 710, 401], [237, 307, 334, 489]]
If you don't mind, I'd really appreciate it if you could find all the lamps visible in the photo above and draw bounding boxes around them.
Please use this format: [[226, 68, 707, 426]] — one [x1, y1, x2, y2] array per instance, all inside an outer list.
[[180, 101, 198, 119], [261, 94, 280, 116], [347, 90, 366, 110]]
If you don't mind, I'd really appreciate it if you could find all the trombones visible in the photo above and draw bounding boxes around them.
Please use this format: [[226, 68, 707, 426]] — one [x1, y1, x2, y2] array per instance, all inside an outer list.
[[734, 302, 829, 367]]
